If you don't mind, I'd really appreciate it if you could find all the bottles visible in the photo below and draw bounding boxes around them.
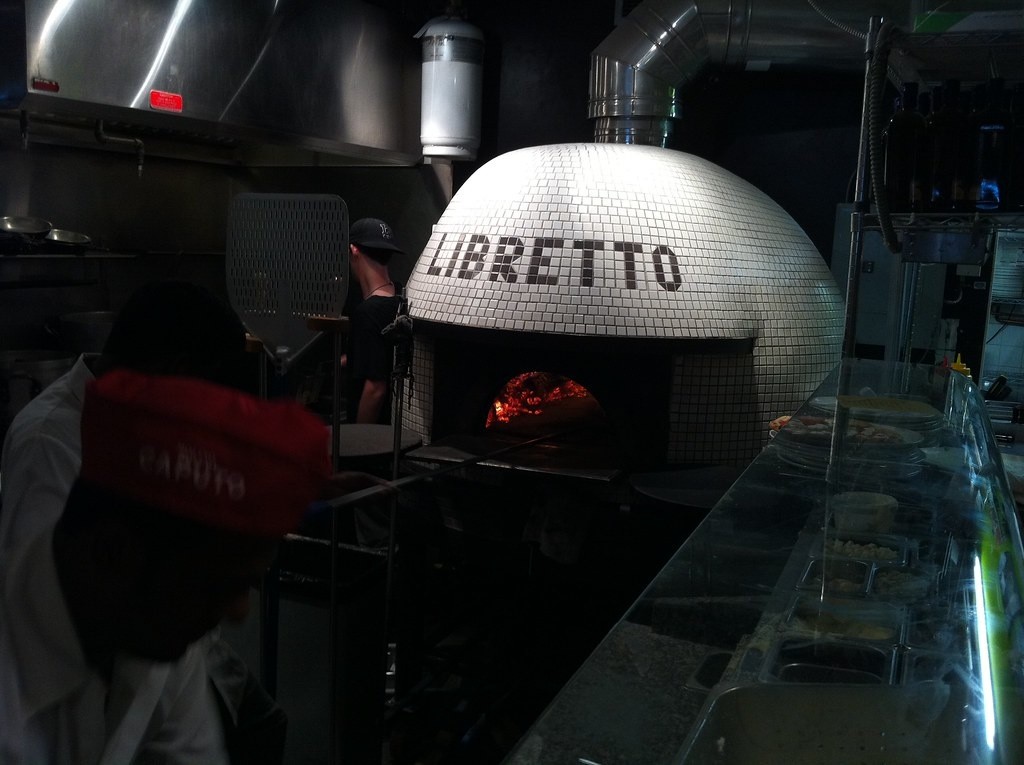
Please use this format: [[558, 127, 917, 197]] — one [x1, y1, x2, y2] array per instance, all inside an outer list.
[[951, 367, 973, 434], [941, 353, 967, 430]]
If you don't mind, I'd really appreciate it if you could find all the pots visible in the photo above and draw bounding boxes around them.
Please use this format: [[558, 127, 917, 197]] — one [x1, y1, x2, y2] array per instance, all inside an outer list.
[[44, 228, 110, 253], [0, 215, 52, 246]]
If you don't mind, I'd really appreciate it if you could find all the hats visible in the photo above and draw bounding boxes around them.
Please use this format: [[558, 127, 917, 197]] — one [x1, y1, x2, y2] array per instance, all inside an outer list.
[[349, 217, 406, 255]]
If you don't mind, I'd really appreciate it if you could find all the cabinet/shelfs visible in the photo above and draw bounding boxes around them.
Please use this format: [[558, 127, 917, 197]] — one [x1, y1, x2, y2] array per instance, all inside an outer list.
[[495, 358, 1022, 765]]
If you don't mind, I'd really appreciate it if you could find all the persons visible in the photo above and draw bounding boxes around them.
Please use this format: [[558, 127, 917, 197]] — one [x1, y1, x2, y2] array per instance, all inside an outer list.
[[321, 218, 416, 577], [0, 366, 332, 764], [0, 290, 250, 583]]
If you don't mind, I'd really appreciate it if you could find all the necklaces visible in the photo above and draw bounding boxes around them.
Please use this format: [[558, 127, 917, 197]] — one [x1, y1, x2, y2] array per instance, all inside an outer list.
[[362, 281, 396, 299]]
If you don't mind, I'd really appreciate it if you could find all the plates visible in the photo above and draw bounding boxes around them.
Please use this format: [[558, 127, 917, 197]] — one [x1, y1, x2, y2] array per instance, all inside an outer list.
[[767, 392, 947, 483]]
